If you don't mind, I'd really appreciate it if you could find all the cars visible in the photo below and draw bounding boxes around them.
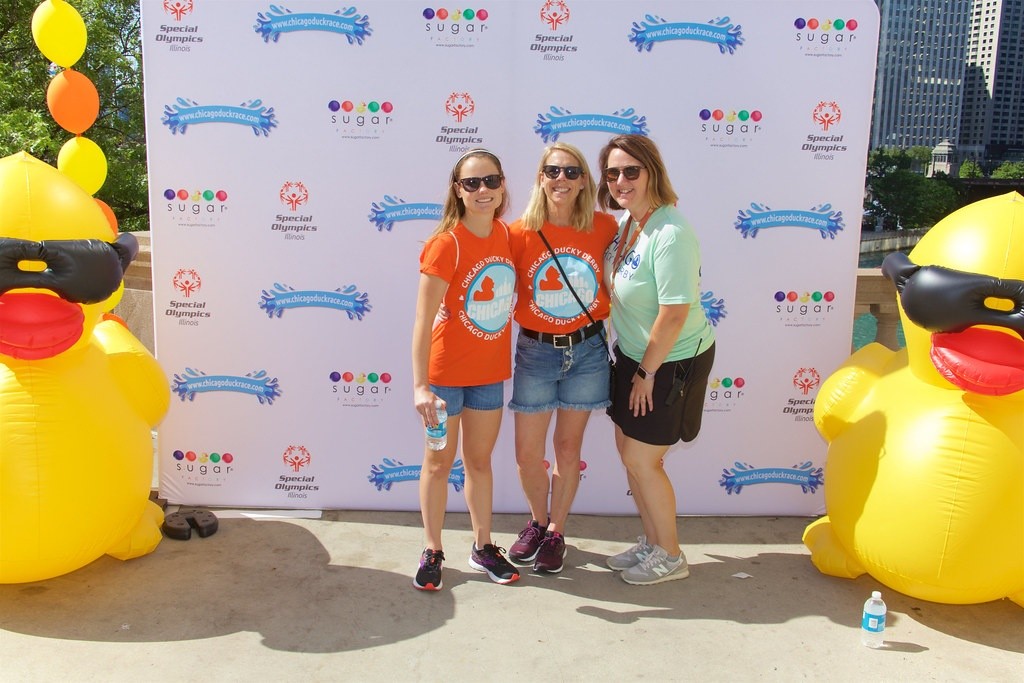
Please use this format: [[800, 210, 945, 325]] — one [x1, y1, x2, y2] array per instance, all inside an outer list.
[[862, 210, 898, 231]]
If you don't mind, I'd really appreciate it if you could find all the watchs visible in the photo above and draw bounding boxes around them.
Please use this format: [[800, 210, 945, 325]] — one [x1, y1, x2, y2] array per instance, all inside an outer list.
[[635, 365, 656, 379]]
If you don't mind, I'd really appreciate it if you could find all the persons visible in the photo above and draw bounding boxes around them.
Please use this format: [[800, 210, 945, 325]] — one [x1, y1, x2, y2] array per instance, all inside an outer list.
[[596, 136, 717, 586], [411, 148, 521, 591], [437, 143, 618, 575]]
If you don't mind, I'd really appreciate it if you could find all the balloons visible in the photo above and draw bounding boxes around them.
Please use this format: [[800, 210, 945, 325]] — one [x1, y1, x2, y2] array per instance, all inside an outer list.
[[46, 70, 99, 137], [94, 196, 123, 237], [56, 137, 107, 198], [31, 1, 88, 70]]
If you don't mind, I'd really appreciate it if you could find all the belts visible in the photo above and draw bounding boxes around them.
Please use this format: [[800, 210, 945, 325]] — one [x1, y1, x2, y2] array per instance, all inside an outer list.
[[517, 318, 604, 347]]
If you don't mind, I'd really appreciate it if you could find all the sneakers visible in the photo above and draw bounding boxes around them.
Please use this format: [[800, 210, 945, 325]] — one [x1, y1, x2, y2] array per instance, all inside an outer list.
[[413, 548, 446, 592], [468, 540, 521, 585], [620, 545, 689, 585], [606, 536, 658, 569], [533, 530, 567, 573], [508, 516, 551, 562]]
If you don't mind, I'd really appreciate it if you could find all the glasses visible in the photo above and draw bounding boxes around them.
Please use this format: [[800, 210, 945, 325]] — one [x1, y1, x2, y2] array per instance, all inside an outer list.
[[541, 165, 586, 180], [602, 166, 647, 183], [458, 175, 505, 192]]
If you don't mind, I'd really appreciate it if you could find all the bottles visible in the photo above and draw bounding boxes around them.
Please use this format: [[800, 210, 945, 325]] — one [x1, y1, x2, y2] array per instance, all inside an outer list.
[[860, 591, 887, 648], [425, 400, 448, 451]]
